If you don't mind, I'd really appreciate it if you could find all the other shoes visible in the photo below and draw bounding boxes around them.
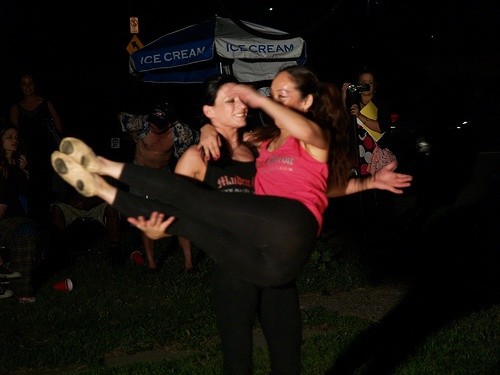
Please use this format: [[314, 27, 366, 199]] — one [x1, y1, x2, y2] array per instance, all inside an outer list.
[[186, 268, 195, 273], [148, 265, 157, 273], [16, 296, 35, 304], [0, 288, 13, 298], [51, 277, 73, 292], [50, 137, 97, 198], [111, 244, 125, 267]]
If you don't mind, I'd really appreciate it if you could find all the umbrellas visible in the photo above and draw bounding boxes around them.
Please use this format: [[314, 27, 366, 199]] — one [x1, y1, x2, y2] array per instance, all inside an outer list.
[[128, 13, 307, 84]]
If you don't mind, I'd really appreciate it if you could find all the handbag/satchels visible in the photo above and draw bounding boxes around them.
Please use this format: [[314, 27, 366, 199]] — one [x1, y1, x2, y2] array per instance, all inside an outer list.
[[367, 147, 397, 174]]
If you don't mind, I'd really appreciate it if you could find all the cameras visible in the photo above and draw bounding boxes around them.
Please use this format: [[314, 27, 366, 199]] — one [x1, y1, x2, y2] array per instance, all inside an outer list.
[[348, 84, 370, 92]]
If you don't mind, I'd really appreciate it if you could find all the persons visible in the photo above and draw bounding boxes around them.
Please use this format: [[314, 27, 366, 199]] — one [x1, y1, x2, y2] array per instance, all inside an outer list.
[[51, 66, 413, 375], [0, 73, 203, 304]]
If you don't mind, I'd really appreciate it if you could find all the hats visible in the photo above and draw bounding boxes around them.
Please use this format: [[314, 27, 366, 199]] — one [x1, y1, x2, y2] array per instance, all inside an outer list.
[[146, 102, 171, 129]]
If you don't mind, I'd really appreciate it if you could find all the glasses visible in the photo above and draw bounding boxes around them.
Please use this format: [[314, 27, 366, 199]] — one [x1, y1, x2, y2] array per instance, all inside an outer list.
[[365, 81, 374, 86]]
